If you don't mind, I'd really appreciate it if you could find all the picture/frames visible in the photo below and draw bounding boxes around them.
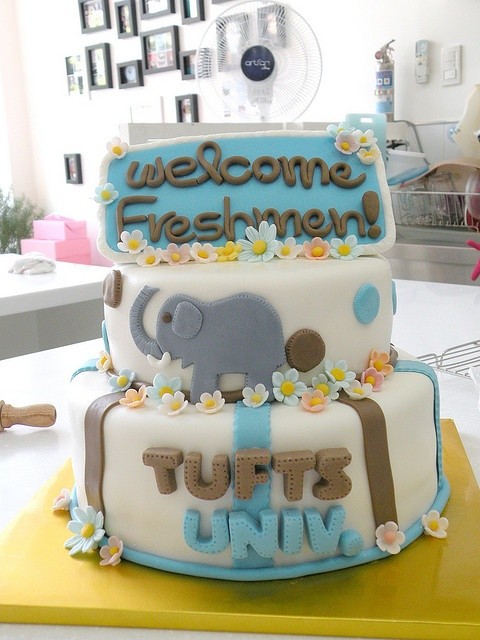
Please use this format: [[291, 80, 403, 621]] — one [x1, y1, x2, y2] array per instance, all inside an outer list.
[[256, 4, 287, 50], [139, 25, 181, 76], [179, 49, 210, 80], [174, 93, 200, 123], [140, 0, 176, 20], [114, 0, 138, 40], [215, 12, 250, 73], [63, 153, 83, 184], [115, 60, 144, 90], [84, 42, 114, 91], [62, 51, 88, 98], [77, 0, 112, 34], [179, 0, 205, 25]]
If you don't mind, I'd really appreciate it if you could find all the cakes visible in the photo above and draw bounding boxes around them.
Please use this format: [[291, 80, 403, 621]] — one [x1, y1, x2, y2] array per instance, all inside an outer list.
[[52, 125, 451, 583]]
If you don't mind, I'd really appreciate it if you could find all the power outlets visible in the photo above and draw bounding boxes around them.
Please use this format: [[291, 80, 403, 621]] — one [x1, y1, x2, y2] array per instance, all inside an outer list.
[[415, 40, 430, 84]]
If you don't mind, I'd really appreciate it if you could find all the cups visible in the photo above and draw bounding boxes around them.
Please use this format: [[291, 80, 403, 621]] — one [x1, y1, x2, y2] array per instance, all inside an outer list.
[[397, 178, 436, 226], [428, 171, 464, 225]]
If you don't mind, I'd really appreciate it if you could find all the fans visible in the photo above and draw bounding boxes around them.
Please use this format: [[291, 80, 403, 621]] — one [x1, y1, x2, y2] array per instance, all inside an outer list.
[[193, 1, 323, 123]]
